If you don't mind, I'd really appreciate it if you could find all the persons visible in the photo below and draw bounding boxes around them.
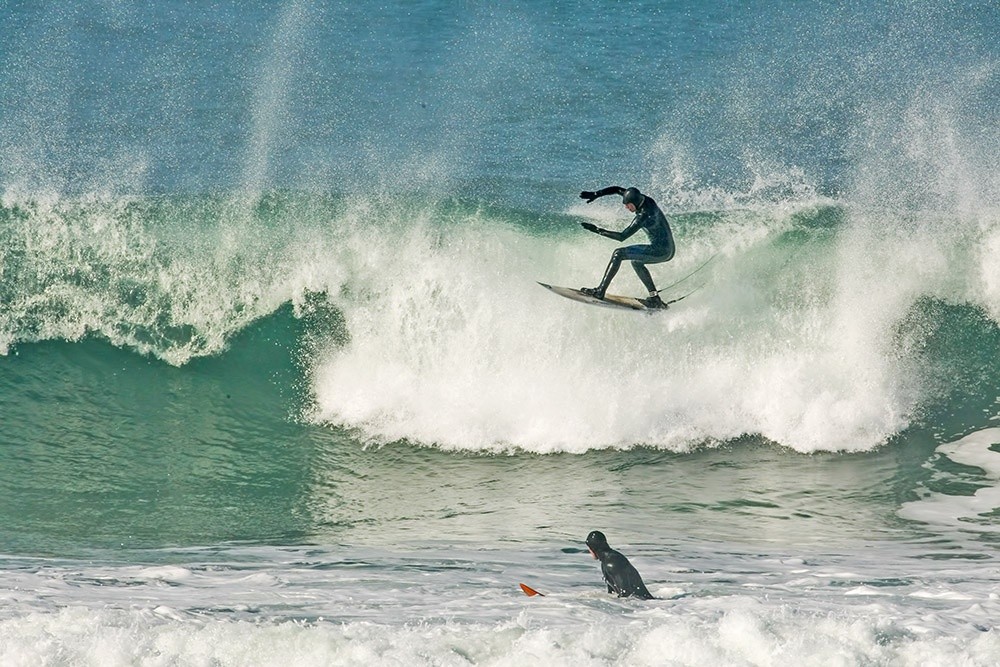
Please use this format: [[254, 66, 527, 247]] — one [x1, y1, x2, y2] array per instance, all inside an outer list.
[[578, 186, 677, 307], [584, 529, 655, 601]]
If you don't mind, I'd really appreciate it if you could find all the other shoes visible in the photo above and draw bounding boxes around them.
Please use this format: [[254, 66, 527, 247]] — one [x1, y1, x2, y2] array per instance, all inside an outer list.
[[581, 288, 606, 300], [635, 296, 663, 308]]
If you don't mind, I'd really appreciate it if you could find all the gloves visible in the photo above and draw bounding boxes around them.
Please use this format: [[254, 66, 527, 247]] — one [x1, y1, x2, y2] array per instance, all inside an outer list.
[[581, 222, 596, 232], [579, 191, 597, 203]]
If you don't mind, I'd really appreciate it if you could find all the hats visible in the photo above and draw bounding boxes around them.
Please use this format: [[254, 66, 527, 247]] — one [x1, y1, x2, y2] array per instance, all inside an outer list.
[[622, 186, 642, 208]]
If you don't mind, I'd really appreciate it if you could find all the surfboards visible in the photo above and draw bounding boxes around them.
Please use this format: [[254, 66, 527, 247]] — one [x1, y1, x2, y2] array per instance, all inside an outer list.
[[536, 282, 670, 311]]
